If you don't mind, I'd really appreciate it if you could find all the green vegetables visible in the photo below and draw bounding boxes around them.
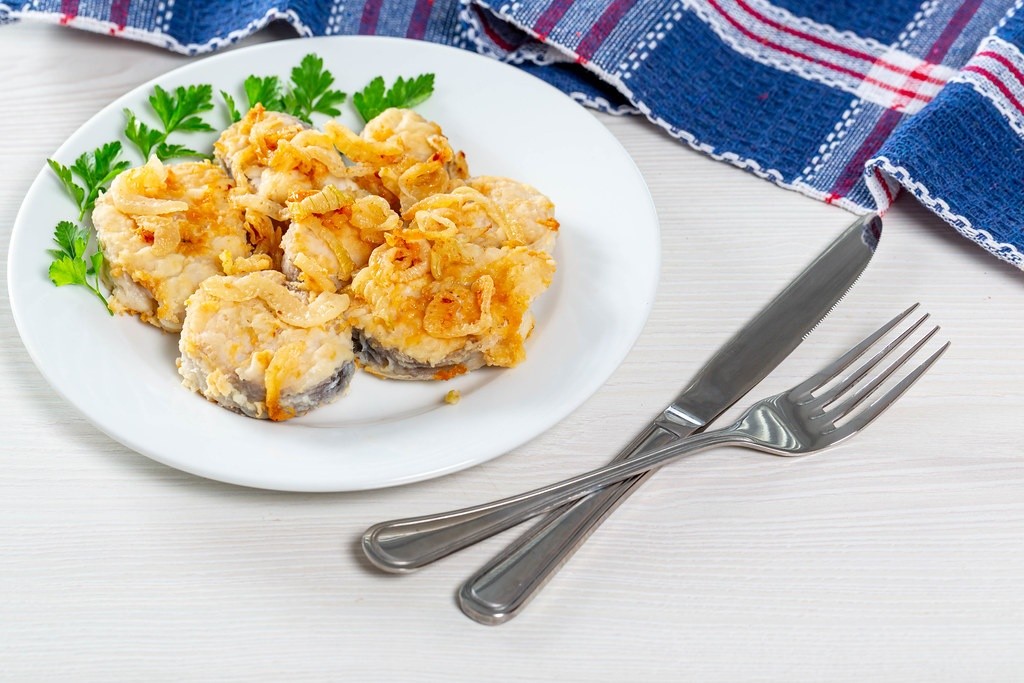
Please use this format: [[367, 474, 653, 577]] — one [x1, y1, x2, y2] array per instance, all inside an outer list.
[[46, 54, 435, 315]]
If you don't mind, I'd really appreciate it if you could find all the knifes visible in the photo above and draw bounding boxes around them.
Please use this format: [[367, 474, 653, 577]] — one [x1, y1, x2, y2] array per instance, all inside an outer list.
[[455, 214, 884, 627]]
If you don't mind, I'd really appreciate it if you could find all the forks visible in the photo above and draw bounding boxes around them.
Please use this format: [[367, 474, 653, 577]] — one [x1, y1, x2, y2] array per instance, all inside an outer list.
[[361, 302, 952, 576]]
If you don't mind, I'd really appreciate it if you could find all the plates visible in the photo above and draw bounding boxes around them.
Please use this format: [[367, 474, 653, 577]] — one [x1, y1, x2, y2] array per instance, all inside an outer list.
[[4, 34, 661, 496]]
